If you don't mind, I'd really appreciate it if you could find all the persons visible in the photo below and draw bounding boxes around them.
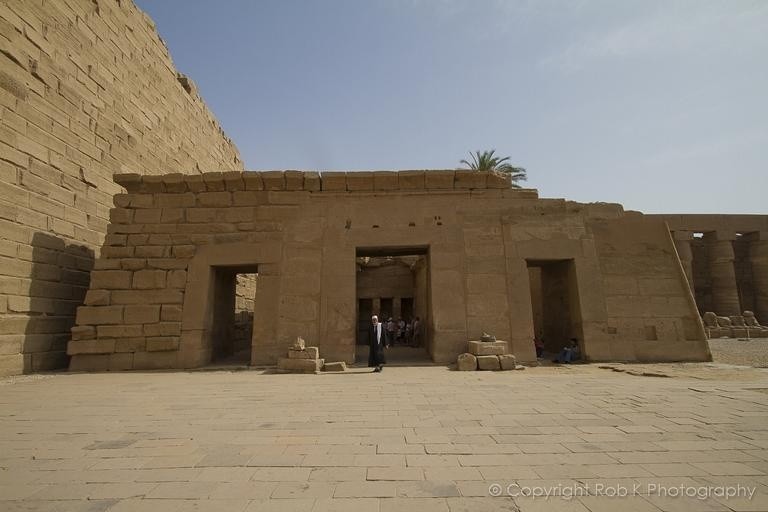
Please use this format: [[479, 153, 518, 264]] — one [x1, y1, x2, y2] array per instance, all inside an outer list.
[[368, 315, 386, 372], [382, 315, 421, 349], [535, 334, 545, 358], [552, 338, 580, 364]]
